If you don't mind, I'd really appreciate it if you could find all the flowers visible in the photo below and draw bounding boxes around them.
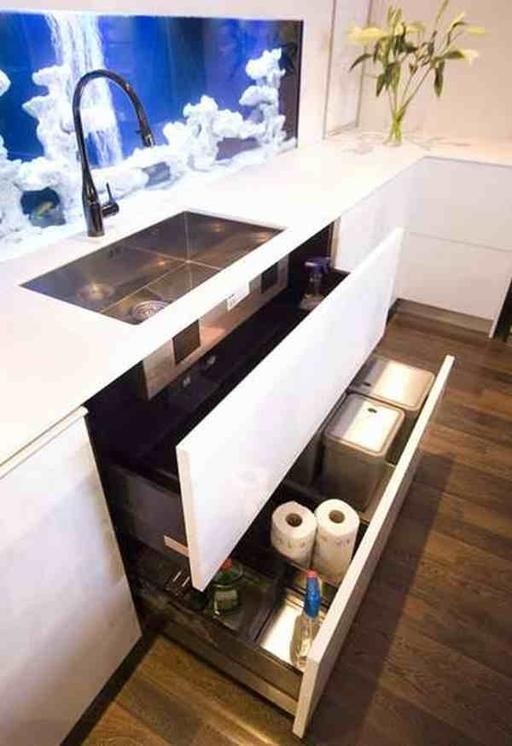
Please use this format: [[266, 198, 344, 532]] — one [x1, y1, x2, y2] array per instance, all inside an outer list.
[[348, 0, 486, 148]]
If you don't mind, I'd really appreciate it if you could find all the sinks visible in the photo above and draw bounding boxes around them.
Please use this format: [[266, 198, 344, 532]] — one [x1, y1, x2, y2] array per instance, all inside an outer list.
[[17, 207, 289, 402]]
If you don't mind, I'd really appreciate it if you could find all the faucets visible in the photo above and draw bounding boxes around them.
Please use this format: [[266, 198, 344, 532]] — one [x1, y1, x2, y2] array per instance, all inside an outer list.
[[70, 69, 155, 236]]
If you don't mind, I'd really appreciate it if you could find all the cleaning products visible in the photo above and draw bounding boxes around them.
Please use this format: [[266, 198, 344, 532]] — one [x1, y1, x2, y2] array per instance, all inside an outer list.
[[289, 571, 321, 674], [299, 254, 331, 312]]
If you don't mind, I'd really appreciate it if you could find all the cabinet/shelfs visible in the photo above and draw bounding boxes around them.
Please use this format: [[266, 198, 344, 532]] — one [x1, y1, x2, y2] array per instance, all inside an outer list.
[[0, 158, 456, 746], [391, 158, 511, 341]]
